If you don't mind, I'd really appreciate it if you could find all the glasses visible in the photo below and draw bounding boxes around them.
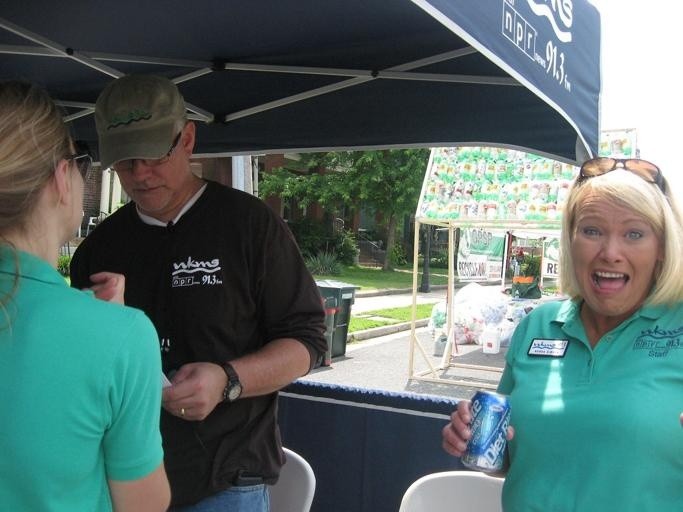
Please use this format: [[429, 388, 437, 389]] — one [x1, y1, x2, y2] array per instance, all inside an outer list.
[[61, 150, 94, 184], [107, 118, 191, 172], [570, 156, 664, 193]]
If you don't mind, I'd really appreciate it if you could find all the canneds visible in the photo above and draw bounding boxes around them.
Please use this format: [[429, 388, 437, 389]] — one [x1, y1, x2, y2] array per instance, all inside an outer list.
[[458, 389, 510, 471]]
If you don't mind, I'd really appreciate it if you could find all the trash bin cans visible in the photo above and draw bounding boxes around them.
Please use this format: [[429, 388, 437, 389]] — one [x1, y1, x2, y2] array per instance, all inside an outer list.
[[315, 280, 363, 366]]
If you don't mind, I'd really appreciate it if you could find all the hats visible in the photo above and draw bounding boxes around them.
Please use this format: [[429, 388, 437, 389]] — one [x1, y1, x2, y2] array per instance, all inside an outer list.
[[90, 71, 189, 172]]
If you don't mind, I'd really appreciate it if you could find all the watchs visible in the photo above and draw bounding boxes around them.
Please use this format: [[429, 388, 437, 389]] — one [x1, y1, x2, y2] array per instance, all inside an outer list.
[[223, 361, 243, 408]]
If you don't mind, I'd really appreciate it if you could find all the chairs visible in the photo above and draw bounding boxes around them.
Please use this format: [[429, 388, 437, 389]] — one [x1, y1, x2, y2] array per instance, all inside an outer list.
[[398, 470, 506, 512], [271, 443, 317, 512]]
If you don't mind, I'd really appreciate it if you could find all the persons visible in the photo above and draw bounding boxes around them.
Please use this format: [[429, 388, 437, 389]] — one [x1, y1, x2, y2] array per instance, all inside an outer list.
[[0, 77, 174, 511], [441, 153, 682, 512], [68, 72, 328, 512]]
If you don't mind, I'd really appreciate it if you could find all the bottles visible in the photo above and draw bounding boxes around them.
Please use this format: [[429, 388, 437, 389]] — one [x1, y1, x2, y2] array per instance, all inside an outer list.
[[480, 322, 500, 354]]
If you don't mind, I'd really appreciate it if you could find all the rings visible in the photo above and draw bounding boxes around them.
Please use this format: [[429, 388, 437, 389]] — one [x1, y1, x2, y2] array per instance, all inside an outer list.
[[179, 408, 186, 416]]
